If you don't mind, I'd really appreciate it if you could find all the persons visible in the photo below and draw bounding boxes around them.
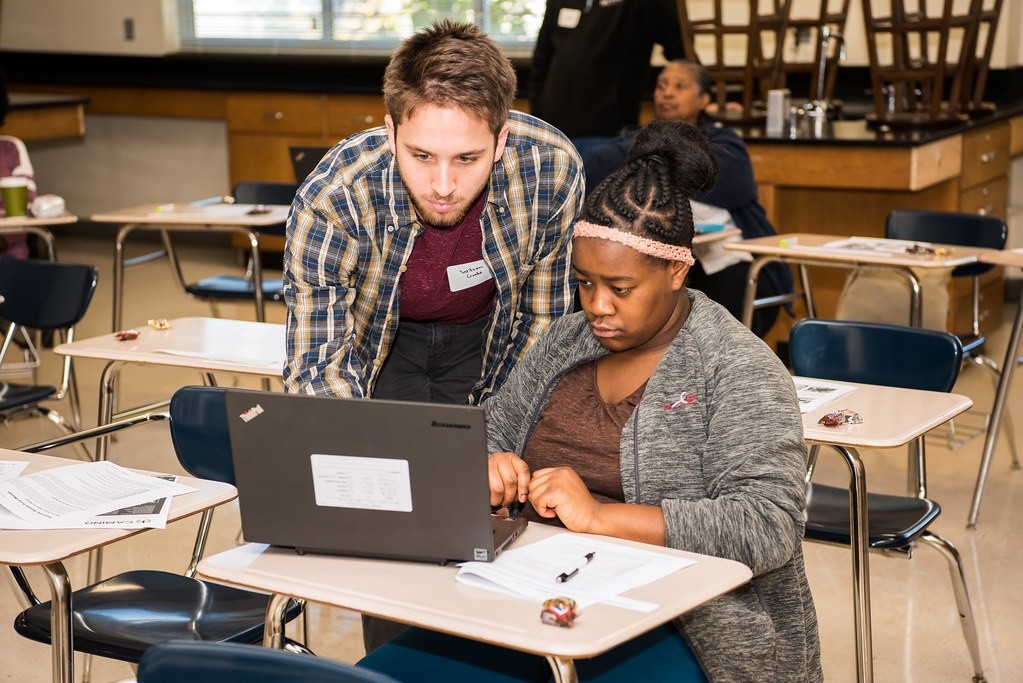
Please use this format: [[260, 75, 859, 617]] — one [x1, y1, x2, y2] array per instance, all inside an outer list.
[[353, 119, 823, 683], [525, 0, 743, 140], [654, 61, 794, 340], [282, 20, 586, 657]]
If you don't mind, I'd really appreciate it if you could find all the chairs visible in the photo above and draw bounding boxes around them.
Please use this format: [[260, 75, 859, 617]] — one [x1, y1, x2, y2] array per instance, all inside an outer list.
[[10, 384, 308, 683], [886, 209, 1008, 452], [136, 639, 397, 683], [187, 181, 300, 385], [788, 317, 987, 683], [0, 253, 99, 463]]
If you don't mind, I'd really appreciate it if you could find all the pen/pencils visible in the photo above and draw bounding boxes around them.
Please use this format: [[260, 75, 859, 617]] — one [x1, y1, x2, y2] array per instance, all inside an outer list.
[[779, 237, 798, 248], [557, 551, 597, 583]]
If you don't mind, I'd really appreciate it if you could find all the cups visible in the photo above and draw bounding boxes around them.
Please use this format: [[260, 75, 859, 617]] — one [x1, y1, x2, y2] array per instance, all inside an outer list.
[[0, 176, 27, 219]]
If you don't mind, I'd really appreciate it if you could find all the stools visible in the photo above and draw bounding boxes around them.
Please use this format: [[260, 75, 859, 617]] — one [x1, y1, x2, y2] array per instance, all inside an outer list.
[[675, 0, 1003, 133]]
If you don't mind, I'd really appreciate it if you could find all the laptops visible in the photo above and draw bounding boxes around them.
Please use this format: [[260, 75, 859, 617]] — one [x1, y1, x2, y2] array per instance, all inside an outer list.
[[224, 388, 527, 566]]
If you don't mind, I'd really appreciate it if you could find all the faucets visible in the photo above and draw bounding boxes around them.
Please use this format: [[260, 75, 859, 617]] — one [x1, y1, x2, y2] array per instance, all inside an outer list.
[[815, 26, 848, 102]]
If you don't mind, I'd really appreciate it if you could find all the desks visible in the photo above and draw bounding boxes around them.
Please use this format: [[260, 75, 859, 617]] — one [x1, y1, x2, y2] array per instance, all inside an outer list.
[[0, 448, 238, 683], [793, 375, 973, 683], [51, 315, 287, 587], [91, 200, 292, 445], [0, 207, 82, 431], [964, 250, 1023, 530], [0, 93, 94, 144], [725, 231, 995, 497], [196, 510, 754, 683]]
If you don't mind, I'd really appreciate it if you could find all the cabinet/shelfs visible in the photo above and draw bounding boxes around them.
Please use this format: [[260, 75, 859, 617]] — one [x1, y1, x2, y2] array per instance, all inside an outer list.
[[224, 91, 532, 267], [731, 105, 1023, 354]]
